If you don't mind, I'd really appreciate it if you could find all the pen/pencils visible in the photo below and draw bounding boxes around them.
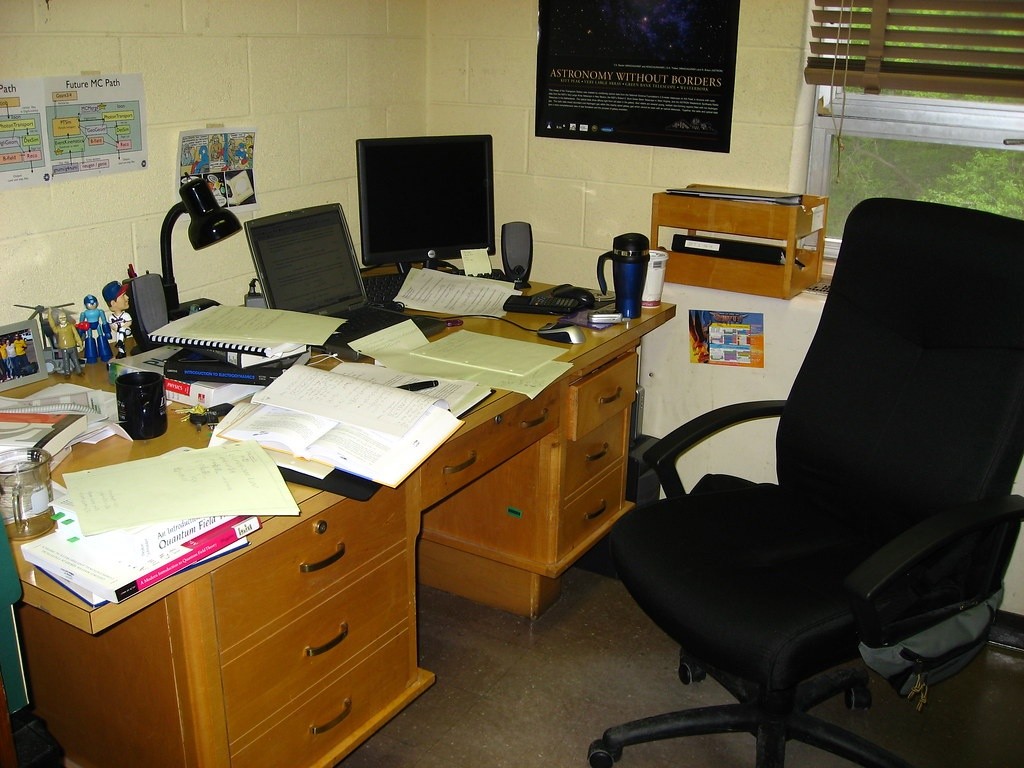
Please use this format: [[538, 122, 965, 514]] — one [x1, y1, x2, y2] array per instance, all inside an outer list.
[[439, 319, 465, 326], [395, 380, 440, 395]]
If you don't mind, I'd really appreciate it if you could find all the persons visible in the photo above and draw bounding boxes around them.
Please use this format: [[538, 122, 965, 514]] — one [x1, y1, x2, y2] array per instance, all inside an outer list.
[[0, 333, 31, 381], [102, 280, 133, 360], [47, 307, 85, 380], [76, 294, 112, 364]]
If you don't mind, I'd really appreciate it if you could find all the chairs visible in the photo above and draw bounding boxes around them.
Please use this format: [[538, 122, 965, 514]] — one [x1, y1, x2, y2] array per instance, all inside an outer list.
[[585, 199, 1024, 768]]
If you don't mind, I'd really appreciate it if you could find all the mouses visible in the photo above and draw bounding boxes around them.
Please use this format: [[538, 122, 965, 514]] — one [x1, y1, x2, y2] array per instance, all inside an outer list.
[[552, 287, 596, 306], [537, 322, 586, 344]]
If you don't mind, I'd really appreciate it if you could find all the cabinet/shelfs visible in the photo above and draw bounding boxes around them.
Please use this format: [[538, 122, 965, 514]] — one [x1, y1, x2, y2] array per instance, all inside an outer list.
[[652, 182, 828, 299]]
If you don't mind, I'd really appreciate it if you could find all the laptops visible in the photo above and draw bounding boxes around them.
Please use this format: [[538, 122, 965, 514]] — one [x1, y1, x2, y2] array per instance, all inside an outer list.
[[245, 203, 447, 360]]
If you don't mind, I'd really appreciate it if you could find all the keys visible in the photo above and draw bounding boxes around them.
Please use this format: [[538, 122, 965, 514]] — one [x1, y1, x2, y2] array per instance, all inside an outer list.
[[174, 405, 219, 431]]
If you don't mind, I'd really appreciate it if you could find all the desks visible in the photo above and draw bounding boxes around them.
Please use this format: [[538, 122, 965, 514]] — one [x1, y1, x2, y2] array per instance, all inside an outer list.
[[0, 266, 677, 767]]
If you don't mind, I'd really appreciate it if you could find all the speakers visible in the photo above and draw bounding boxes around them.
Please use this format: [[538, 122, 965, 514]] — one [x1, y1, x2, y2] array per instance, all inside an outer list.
[[501, 222, 534, 290], [122, 273, 171, 357]]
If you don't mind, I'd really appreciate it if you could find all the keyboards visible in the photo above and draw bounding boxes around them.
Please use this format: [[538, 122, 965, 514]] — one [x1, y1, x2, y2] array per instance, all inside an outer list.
[[363, 268, 507, 307]]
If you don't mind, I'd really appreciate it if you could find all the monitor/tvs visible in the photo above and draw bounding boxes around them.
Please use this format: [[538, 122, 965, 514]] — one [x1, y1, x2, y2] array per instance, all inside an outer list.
[[355, 134, 496, 273]]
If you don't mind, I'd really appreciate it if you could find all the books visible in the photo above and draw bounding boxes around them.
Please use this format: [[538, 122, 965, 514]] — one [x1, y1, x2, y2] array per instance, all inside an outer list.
[[19, 446, 261, 609], [0, 396, 87, 474], [108, 305, 313, 409], [217, 358, 497, 503]]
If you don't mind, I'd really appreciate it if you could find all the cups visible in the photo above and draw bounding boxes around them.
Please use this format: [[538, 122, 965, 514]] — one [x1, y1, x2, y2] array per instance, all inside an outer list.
[[1, 447, 56, 540], [115, 372, 168, 439], [596, 233, 651, 318], [641, 250, 669, 308]]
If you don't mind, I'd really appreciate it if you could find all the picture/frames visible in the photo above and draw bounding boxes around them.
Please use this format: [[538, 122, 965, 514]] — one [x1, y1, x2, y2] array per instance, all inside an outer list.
[[0, 319, 49, 394]]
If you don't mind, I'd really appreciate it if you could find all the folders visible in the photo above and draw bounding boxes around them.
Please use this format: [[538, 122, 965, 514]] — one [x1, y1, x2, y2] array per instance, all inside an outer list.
[[671, 232, 788, 267]]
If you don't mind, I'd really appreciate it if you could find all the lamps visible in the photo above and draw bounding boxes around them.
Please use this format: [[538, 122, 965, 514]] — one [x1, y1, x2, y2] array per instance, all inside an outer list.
[[160, 179, 240, 317]]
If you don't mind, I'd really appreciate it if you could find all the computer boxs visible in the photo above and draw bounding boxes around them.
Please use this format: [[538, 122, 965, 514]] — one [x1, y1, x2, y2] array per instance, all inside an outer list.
[[577, 434, 663, 580]]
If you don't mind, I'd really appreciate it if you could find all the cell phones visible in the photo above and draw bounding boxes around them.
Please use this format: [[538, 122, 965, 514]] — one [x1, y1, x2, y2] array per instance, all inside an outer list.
[[502, 295, 578, 315], [587, 311, 631, 325]]
